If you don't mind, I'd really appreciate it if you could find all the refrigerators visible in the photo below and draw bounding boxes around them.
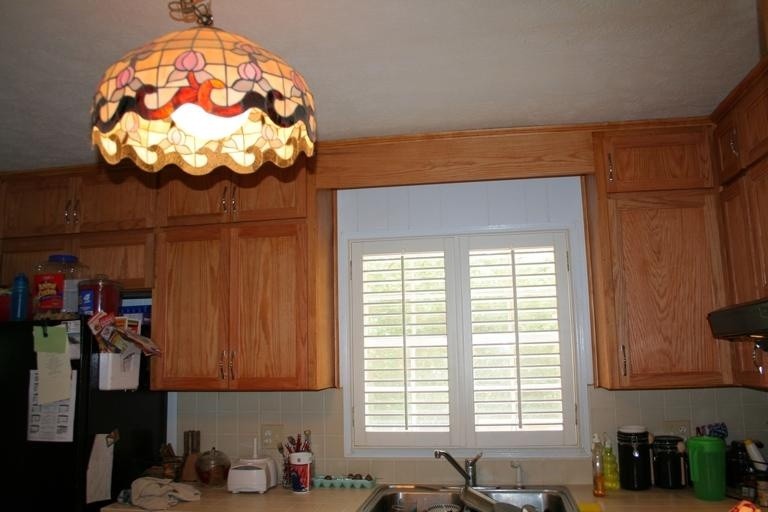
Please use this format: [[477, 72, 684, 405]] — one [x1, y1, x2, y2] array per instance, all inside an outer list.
[[0, 320, 169, 511]]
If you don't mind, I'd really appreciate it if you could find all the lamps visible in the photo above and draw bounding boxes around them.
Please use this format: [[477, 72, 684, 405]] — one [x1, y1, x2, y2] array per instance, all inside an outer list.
[[90, 1, 318, 180]]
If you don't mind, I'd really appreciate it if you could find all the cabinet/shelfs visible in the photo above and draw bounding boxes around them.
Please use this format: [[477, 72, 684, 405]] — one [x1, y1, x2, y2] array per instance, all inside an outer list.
[[0, 161, 153, 238], [150, 229, 343, 394], [153, 143, 342, 227], [712, 55, 768, 392], [578, 120, 735, 389], [0, 239, 150, 288]]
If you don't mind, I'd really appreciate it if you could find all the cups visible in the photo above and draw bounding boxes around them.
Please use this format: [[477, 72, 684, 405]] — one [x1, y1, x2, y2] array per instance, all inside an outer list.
[[290, 452, 314, 494], [315, 478, 376, 489], [161, 456, 183, 484], [281, 464, 291, 489], [687, 436, 726, 501], [726, 440, 768, 506], [182, 454, 201, 483]]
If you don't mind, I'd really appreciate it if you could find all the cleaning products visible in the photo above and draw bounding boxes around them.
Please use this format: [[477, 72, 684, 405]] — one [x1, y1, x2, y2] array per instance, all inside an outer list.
[[590, 431, 622, 497]]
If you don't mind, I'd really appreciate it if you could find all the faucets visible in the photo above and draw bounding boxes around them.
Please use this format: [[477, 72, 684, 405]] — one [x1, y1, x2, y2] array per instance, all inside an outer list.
[[433, 448, 479, 486], [508, 459, 526, 489]]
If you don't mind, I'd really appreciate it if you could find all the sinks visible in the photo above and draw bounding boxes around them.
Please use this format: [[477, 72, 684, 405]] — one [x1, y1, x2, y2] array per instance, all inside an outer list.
[[470, 487, 572, 510], [366, 486, 470, 510]]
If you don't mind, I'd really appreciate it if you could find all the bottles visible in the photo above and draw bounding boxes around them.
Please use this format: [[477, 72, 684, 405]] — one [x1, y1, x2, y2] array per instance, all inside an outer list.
[[618, 424, 687, 491], [8, 273, 29, 320], [590, 432, 620, 495], [31, 254, 125, 319], [196, 447, 230, 487]]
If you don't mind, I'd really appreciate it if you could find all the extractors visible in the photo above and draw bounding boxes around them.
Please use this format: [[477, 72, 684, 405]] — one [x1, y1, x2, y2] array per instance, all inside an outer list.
[[706, 297, 768, 349]]
